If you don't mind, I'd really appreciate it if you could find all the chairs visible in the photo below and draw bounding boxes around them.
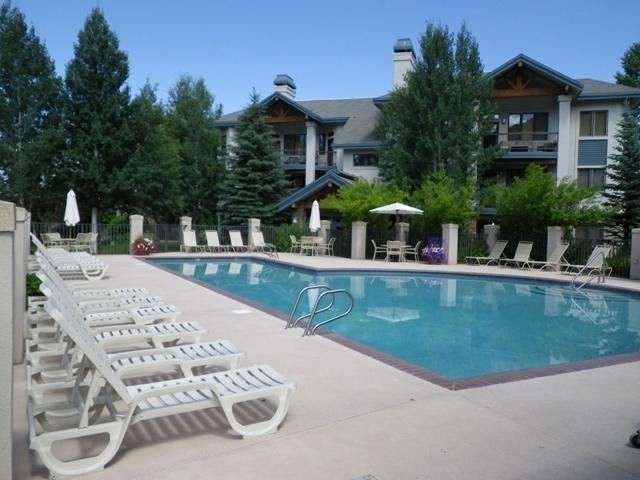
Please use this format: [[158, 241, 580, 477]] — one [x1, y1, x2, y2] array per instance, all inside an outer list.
[[498, 241, 534, 271], [559, 245, 613, 278], [228, 231, 252, 253], [528, 243, 571, 273], [26, 250, 297, 478], [179, 231, 207, 254], [288, 234, 337, 257], [465, 240, 507, 270], [27, 231, 110, 281], [371, 240, 421, 264], [251, 232, 278, 252], [205, 231, 230, 252]]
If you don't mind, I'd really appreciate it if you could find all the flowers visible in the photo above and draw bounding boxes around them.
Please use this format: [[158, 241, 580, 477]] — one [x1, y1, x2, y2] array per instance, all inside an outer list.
[[422, 238, 447, 260]]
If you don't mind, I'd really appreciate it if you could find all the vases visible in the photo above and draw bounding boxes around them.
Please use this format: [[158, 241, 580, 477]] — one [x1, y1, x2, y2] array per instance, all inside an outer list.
[[429, 257, 437, 264]]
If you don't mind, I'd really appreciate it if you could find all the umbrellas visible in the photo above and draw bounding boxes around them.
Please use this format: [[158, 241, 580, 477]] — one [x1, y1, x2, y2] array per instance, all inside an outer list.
[[309, 201, 322, 238], [64, 188, 81, 240], [367, 201, 425, 225]]
